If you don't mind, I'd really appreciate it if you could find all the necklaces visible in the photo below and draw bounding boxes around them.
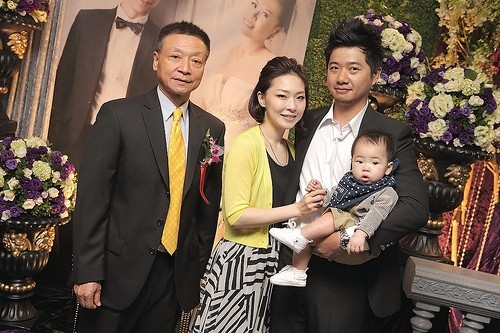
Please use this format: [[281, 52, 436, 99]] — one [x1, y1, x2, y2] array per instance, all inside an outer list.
[[258, 125, 287, 166]]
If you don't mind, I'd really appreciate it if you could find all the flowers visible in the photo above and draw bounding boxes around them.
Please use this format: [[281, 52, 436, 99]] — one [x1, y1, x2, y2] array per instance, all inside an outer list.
[[0, 136, 77, 225], [350, 8, 500, 155], [0, 0, 50, 29], [201, 128, 224, 166]]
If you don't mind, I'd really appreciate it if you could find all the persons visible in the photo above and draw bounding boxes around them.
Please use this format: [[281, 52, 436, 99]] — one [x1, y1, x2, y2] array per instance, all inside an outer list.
[[191, 0, 297, 152], [265, 17, 431, 333], [47, 0, 165, 173], [268, 127, 398, 287], [189, 56, 309, 333], [68, 22, 225, 333]]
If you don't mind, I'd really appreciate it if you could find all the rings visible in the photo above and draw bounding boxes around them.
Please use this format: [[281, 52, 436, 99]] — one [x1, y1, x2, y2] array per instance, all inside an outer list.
[[317, 202, 321, 208]]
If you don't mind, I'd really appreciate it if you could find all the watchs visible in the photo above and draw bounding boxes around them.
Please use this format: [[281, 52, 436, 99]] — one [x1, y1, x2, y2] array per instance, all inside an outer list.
[[339, 228, 351, 251]]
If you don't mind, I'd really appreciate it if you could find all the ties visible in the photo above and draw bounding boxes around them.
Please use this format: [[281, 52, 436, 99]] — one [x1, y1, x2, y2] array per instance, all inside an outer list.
[[161, 107, 187, 257]]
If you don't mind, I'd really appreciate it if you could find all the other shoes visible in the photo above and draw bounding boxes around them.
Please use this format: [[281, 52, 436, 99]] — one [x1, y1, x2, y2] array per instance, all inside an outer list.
[[269, 219, 313, 254], [270, 265, 308, 287]]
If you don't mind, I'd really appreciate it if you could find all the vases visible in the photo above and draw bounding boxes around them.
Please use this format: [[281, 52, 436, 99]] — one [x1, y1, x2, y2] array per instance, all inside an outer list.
[[399, 138, 487, 260], [0, 18, 46, 122], [367, 84, 407, 116], [0, 217, 59, 322]]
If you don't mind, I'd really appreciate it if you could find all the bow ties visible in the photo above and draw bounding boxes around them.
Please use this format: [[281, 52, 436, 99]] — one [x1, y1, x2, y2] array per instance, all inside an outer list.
[[115, 16, 143, 35]]
